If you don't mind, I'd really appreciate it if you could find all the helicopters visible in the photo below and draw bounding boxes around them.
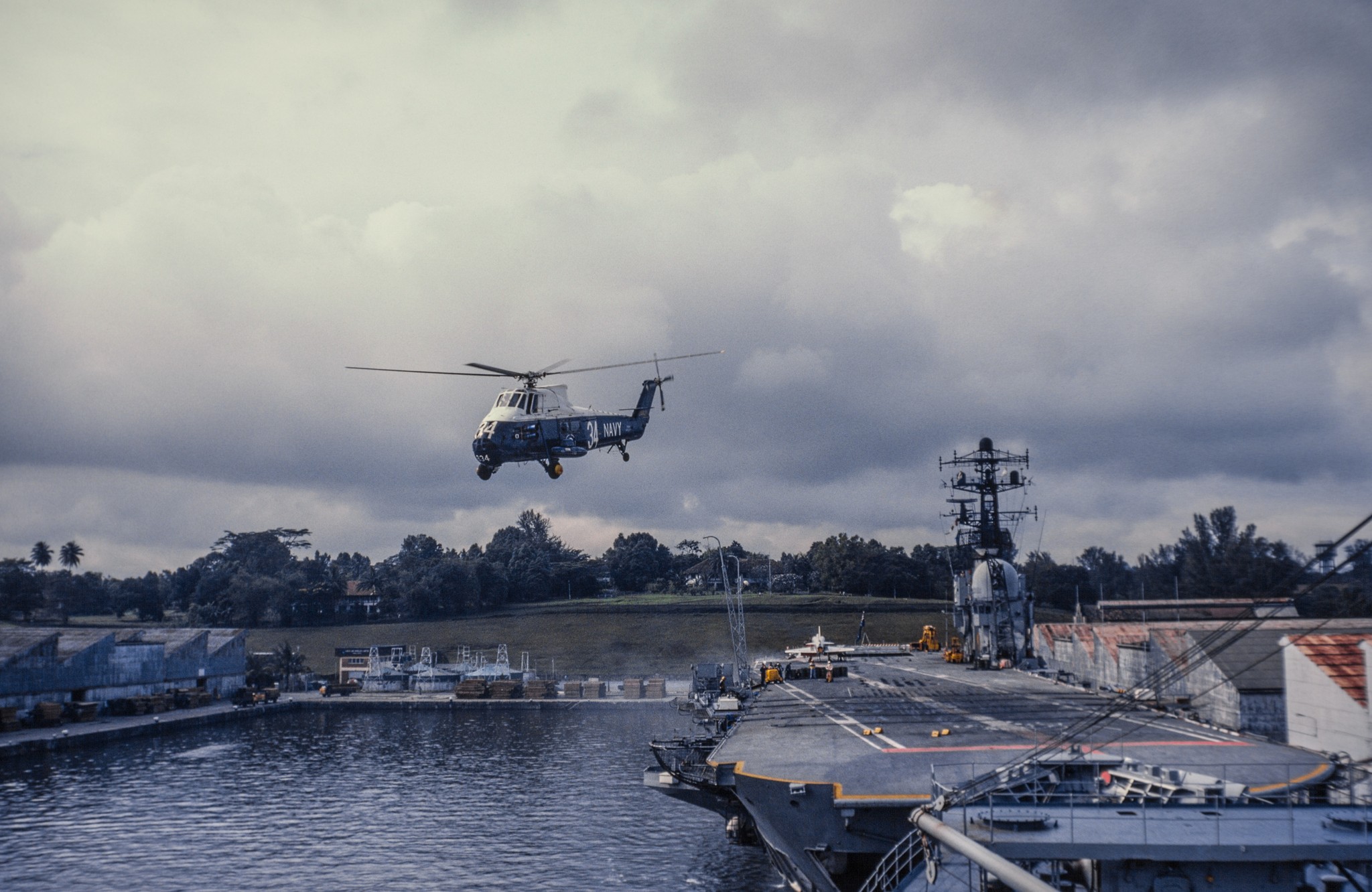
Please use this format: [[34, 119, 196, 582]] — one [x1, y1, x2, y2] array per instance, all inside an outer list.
[[345, 349, 724, 481]]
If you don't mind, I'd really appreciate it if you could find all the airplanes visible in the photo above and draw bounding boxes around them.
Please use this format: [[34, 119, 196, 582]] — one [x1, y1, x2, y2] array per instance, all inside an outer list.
[[785, 625, 855, 663]]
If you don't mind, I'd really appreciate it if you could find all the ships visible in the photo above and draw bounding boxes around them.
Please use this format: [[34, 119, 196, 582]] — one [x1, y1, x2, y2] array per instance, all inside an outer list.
[[643, 438, 1370, 892]]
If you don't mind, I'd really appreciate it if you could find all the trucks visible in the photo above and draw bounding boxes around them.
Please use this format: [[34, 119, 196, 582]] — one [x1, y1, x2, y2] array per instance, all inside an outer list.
[[320, 684, 360, 697], [254, 688, 280, 704]]
